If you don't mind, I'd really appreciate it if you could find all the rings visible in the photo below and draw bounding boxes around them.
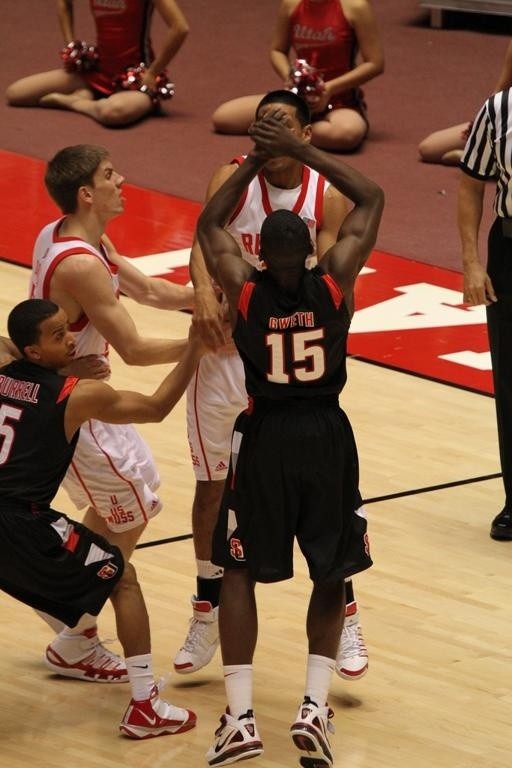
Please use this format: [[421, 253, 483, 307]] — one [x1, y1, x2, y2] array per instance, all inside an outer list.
[[467, 297, 471, 302]]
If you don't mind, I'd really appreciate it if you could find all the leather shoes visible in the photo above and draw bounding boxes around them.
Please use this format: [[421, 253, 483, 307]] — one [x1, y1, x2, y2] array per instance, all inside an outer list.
[[490, 503, 511, 540]]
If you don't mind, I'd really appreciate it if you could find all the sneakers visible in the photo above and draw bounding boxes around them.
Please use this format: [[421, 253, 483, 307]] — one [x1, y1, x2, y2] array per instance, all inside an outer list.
[[206, 705, 264, 768], [43, 627, 129, 683], [289, 695, 335, 768], [118, 685, 197, 739], [173, 595, 220, 674], [337, 600, 369, 680]]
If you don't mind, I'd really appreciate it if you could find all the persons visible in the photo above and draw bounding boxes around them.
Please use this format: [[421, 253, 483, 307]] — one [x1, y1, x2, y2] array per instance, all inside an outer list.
[[212, 0, 384, 151], [173, 90, 369, 678], [7, 0, 190, 126], [31, 144, 233, 682], [0, 299, 231, 742], [453, 87, 512, 539], [195, 104, 385, 768], [417, 40, 512, 165]]
